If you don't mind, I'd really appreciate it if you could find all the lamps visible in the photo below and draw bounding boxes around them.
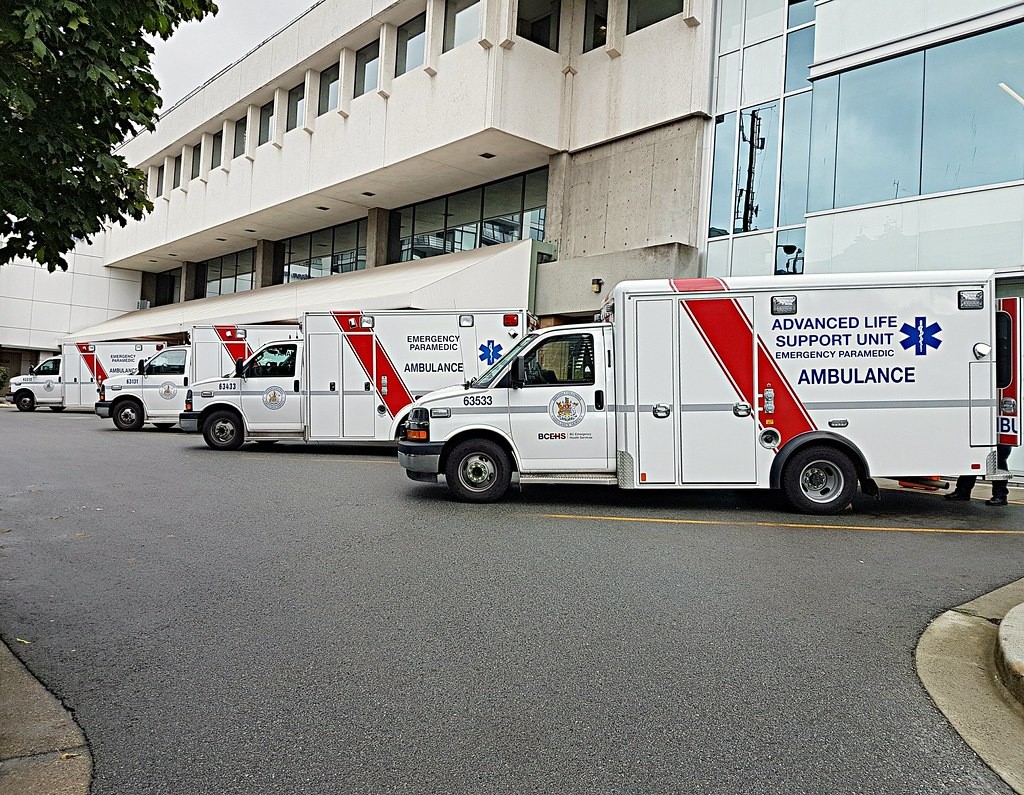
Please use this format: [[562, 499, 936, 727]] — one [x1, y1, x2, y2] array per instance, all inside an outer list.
[[592, 279, 603, 293]]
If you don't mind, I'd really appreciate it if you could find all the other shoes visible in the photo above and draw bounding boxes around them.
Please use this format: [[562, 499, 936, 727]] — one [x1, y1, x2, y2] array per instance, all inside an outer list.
[[985, 495, 1008, 506], [945, 491, 971, 500]]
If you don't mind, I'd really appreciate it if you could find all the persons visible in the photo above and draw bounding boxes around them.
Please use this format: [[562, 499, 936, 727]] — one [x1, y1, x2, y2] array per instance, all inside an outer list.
[[945, 445, 1011, 505]]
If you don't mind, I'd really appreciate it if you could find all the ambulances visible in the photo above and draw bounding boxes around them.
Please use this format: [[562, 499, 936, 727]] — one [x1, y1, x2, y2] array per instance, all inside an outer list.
[[176, 307, 541, 454], [95, 322, 304, 432], [4, 339, 168, 415], [387, 268, 1024, 505]]
